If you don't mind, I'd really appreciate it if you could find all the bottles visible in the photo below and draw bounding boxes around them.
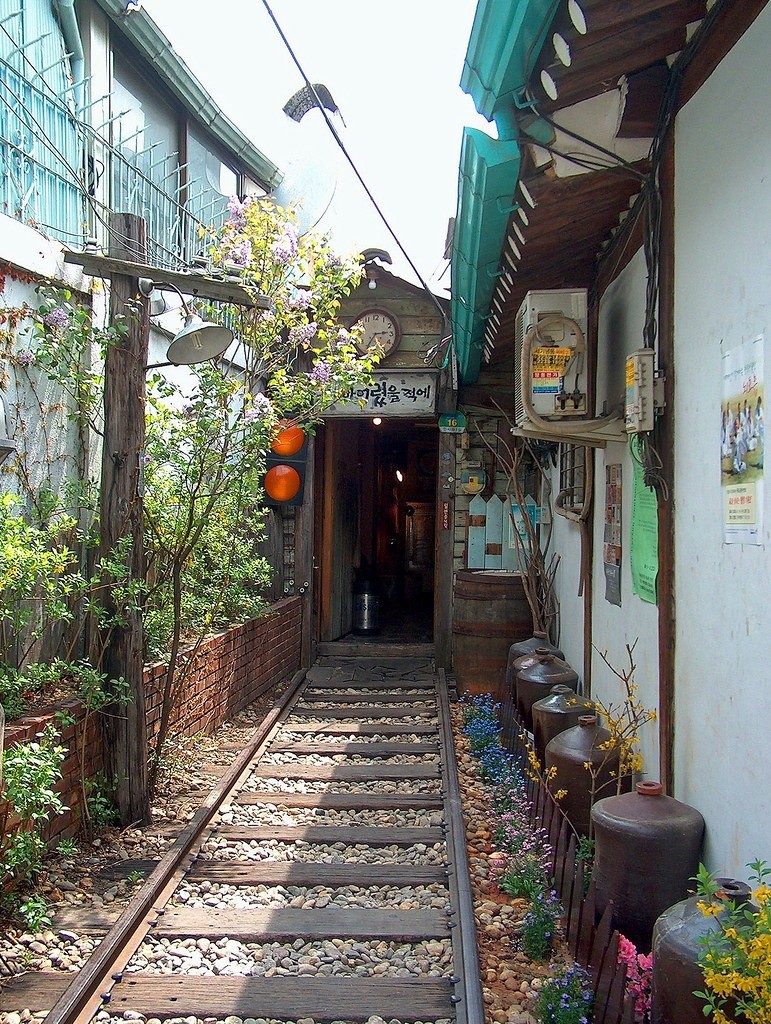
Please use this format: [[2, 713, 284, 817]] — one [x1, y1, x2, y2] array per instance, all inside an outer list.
[[544, 716, 631, 849], [588, 779, 705, 955], [649, 878, 760, 1024], [528, 686, 593, 774], [505, 628, 576, 714]]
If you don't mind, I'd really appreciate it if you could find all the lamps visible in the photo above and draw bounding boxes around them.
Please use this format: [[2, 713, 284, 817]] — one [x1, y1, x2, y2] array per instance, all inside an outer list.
[[138, 271, 234, 368]]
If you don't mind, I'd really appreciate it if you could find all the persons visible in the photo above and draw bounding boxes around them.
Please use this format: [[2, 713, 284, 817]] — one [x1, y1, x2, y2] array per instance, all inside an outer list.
[[719, 393, 763, 475]]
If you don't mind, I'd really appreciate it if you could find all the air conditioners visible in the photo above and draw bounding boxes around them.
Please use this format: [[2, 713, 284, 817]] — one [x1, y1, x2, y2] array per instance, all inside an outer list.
[[510, 287, 592, 425]]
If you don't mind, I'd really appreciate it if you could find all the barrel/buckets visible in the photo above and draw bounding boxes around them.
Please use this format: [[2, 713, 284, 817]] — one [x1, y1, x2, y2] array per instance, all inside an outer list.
[[450, 566, 538, 703], [354, 590, 380, 633]]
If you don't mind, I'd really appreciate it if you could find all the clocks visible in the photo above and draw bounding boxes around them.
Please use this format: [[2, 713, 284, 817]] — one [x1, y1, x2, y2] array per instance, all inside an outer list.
[[351, 306, 402, 359]]
